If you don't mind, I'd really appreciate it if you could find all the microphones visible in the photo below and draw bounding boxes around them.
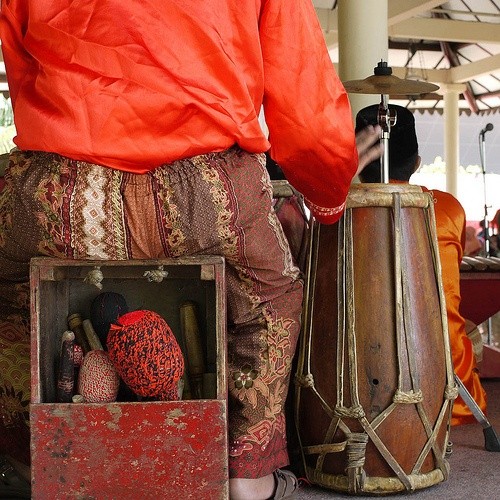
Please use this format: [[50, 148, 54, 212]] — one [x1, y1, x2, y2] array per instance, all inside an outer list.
[[480, 123, 493, 135]]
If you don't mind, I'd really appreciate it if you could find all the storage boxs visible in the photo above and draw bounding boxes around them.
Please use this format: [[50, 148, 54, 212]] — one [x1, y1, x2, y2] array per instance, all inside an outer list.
[[29, 254, 229, 500]]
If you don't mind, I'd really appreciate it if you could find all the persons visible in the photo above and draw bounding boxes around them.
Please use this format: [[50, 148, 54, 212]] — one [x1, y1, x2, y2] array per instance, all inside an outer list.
[[355, 101, 488, 430], [0, 1, 384, 500]]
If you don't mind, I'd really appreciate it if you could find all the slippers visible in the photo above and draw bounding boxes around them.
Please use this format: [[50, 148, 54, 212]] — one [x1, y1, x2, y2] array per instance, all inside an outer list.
[[272, 468, 299, 500]]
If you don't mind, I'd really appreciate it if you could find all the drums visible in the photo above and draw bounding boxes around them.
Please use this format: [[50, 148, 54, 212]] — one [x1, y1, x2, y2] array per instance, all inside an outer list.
[[262, 179, 310, 263], [295, 179, 454, 492]]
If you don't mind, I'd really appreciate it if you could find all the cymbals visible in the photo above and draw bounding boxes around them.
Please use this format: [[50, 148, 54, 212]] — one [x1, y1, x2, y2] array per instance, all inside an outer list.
[[344, 58, 440, 96]]
